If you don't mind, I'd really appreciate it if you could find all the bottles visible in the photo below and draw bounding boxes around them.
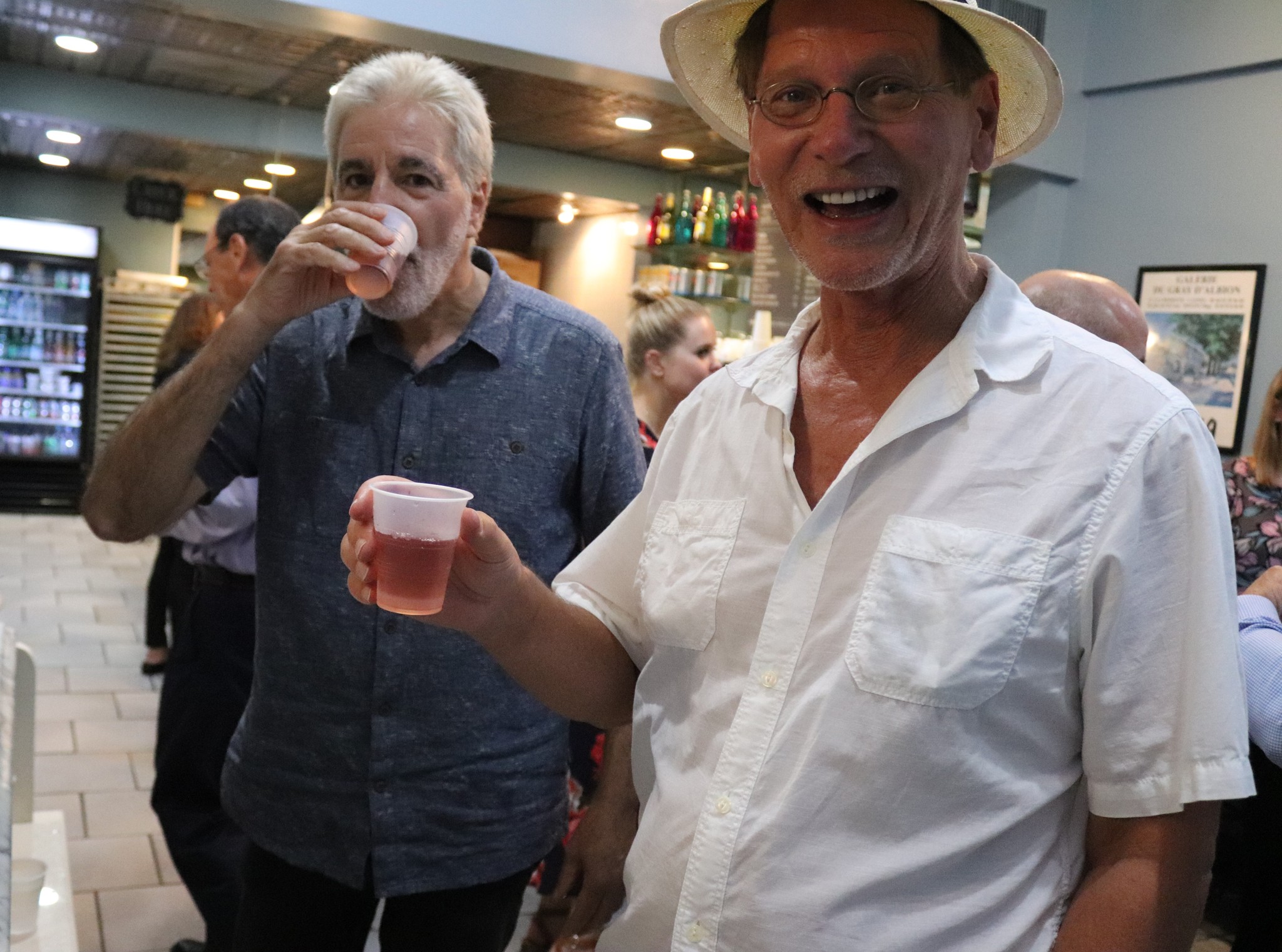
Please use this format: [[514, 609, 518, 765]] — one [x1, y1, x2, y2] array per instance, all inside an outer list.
[[648, 187, 758, 255], [0, 290, 85, 457]]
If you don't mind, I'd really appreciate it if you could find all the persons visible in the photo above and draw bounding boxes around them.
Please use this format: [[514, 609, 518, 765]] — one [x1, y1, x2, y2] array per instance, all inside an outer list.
[[79, 50, 648, 952], [142, 197, 306, 951], [338, 0, 1256, 952], [1020, 270, 1282, 952], [518, 288, 724, 952]]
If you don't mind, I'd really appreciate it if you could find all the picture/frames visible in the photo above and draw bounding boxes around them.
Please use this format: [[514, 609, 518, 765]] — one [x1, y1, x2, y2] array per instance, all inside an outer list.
[[1135, 263, 1268, 455]]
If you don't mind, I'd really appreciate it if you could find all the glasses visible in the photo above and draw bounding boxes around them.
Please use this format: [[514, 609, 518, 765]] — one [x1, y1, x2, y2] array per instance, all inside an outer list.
[[746, 72, 974, 129]]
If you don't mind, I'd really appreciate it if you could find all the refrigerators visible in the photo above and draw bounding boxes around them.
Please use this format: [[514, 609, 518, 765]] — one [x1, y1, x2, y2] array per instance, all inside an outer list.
[[0, 213, 103, 516]]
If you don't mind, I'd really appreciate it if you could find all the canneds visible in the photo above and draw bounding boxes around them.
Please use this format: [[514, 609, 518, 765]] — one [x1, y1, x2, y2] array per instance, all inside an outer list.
[[636, 264, 751, 298]]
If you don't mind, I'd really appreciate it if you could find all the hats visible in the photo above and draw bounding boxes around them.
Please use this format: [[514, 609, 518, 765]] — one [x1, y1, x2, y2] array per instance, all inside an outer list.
[[659, 0, 1064, 175]]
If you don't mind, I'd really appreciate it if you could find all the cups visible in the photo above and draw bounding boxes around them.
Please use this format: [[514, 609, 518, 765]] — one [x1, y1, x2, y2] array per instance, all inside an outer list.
[[368, 481, 474, 616], [342, 203, 418, 300]]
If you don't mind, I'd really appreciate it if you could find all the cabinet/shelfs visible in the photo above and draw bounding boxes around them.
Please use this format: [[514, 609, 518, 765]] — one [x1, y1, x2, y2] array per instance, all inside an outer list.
[[632, 241, 753, 307]]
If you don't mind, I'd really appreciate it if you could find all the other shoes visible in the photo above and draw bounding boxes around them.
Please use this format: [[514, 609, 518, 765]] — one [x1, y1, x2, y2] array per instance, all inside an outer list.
[[143, 646, 173, 674]]
